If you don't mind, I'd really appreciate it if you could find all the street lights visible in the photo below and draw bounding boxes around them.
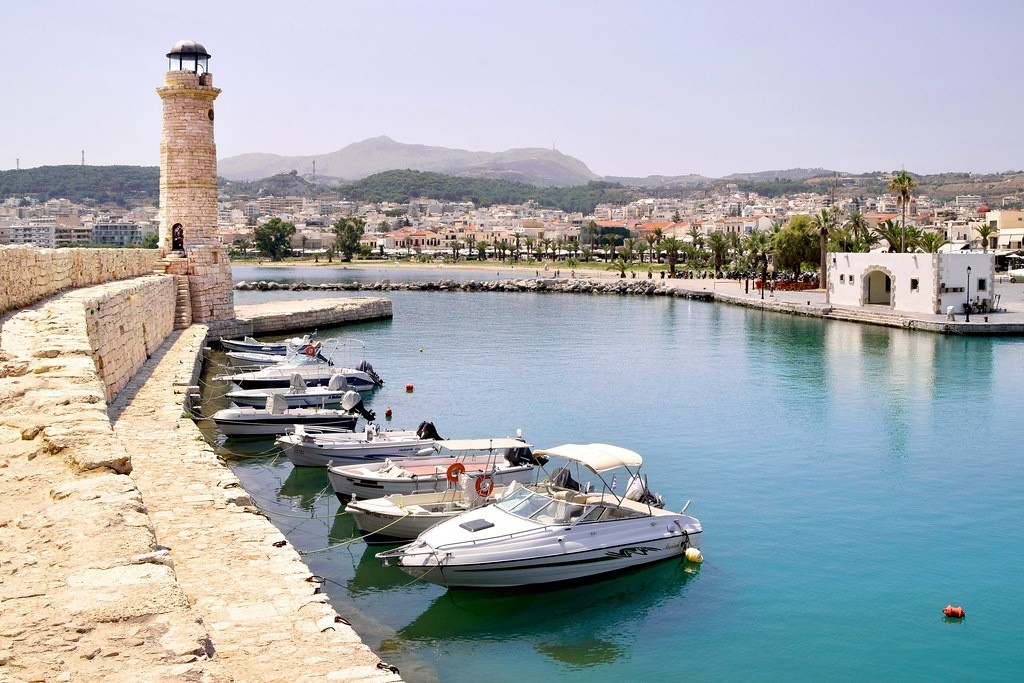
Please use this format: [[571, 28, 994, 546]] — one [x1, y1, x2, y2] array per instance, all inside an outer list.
[[966, 266, 971, 322]]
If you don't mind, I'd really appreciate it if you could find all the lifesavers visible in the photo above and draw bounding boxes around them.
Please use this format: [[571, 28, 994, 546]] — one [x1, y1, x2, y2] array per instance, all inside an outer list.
[[446, 462, 465, 482], [306, 346, 315, 356], [474, 473, 494, 497]]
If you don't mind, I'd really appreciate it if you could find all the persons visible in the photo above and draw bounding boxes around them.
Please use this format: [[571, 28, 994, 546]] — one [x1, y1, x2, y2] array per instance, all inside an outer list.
[[969, 296, 983, 313]]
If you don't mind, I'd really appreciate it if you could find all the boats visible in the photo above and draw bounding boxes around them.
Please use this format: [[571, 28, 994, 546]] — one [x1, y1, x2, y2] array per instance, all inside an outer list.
[[213, 329, 385, 440], [344, 443, 703, 590], [325, 429, 549, 507], [272, 419, 443, 469]]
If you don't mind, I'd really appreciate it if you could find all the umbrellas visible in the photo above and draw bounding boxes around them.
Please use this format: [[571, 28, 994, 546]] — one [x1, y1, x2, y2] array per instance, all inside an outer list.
[[1005, 253, 1021, 263]]
